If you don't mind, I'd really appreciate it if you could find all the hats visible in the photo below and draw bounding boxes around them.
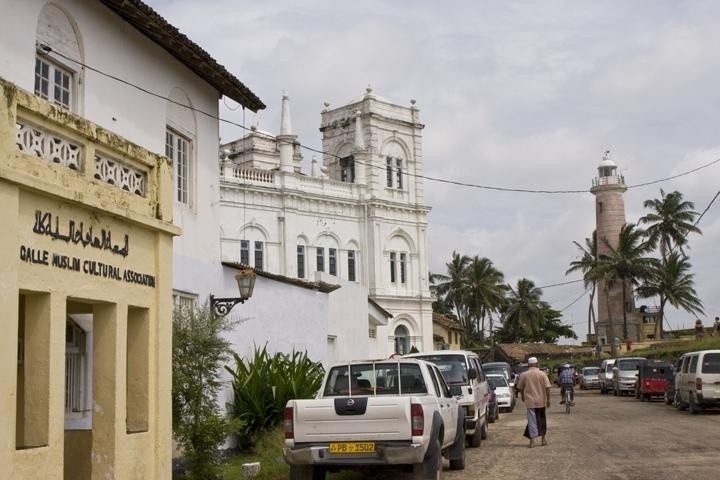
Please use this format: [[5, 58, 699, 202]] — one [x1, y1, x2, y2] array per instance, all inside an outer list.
[[528, 357, 537, 364], [563, 364, 569, 368]]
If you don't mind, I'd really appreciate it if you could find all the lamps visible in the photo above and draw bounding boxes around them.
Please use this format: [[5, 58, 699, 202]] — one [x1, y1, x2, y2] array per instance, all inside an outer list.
[[208, 267, 259, 335]]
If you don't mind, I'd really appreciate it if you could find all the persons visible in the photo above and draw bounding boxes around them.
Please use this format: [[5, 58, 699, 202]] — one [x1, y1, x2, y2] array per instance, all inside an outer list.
[[714, 317, 720, 332], [558, 363, 576, 406], [591, 345, 596, 362], [596, 345, 601, 362], [517, 356, 552, 448], [569, 345, 573, 361], [695, 319, 705, 341], [627, 335, 633, 352]]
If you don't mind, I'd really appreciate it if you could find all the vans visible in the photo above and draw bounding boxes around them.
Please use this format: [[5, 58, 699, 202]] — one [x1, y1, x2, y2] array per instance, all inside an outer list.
[[578, 349, 720, 414]]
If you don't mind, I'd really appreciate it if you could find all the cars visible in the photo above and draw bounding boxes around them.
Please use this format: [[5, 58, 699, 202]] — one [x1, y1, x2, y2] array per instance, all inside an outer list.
[[281, 349, 529, 480], [552, 363, 579, 387]]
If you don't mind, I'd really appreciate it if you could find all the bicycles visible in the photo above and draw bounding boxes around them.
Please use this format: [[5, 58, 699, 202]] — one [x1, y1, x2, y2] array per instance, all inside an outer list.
[[560, 385, 576, 414]]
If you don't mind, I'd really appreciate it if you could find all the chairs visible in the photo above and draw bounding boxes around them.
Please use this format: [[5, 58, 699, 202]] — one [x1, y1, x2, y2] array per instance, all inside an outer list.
[[333, 373, 376, 396]]
